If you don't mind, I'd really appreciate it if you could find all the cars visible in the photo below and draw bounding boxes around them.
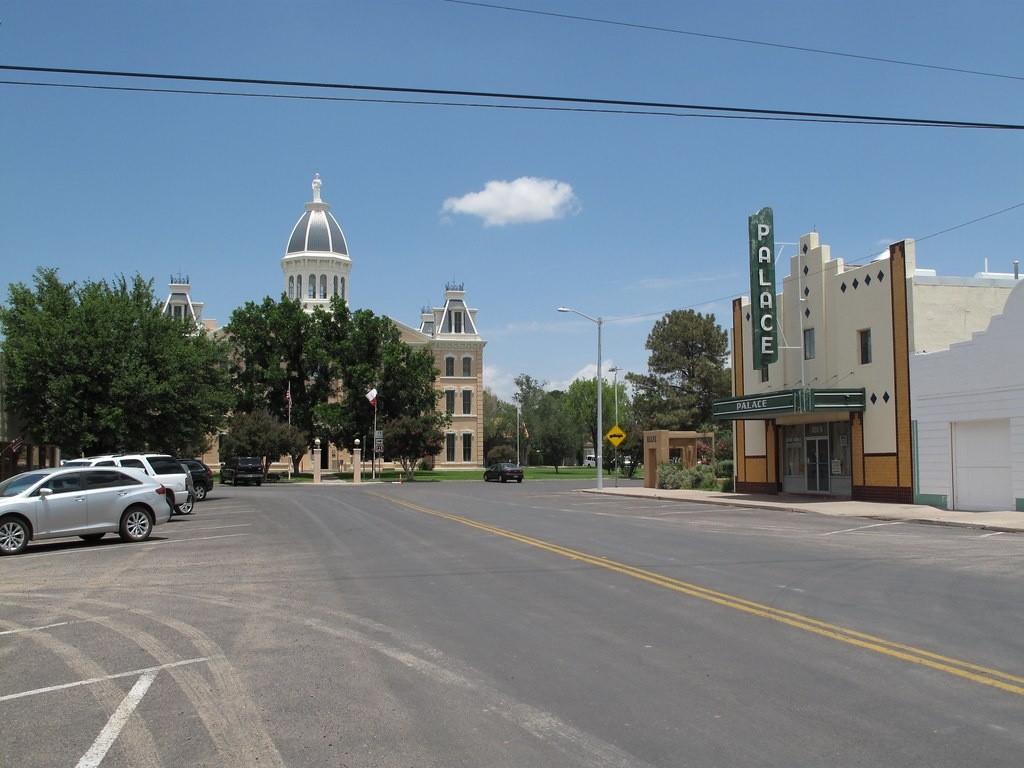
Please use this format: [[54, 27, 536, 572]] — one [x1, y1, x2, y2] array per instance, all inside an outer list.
[[483, 462, 524, 483], [0, 464, 173, 557], [611, 455, 634, 468]]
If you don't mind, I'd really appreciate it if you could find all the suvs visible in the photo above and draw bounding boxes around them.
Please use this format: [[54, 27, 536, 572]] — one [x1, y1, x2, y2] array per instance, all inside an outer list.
[[219, 456, 264, 487], [45, 450, 214, 515]]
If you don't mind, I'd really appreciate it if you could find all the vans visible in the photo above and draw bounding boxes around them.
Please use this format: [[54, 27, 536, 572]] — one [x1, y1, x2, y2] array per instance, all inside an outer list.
[[584, 455, 595, 468]]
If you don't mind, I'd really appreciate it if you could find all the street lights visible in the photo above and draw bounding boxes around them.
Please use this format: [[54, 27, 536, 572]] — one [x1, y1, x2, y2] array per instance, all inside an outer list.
[[556, 305, 604, 492]]
